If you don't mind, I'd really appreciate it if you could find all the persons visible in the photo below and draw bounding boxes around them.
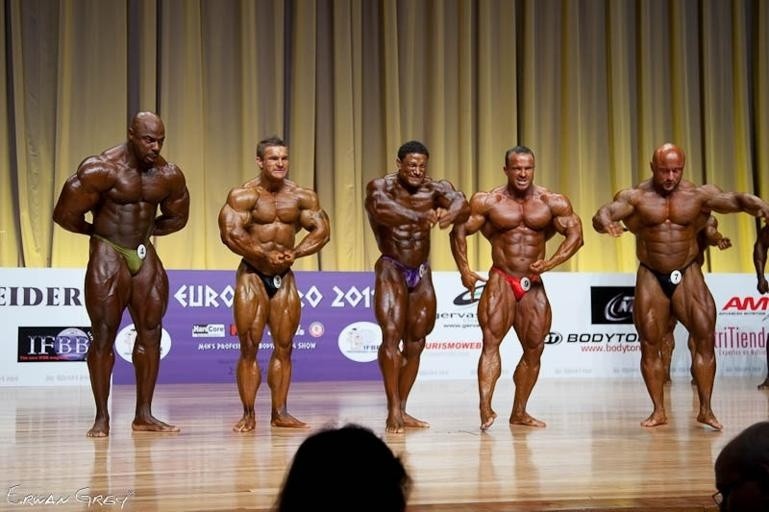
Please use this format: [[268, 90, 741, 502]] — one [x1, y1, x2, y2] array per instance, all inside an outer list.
[[47, 111, 191, 438], [447, 144, 584, 431], [662, 215, 734, 387], [713, 420, 769, 512], [220, 135, 332, 434], [272, 419, 413, 511], [365, 141, 473, 433], [591, 141, 768, 432], [752, 224, 769, 391]]
